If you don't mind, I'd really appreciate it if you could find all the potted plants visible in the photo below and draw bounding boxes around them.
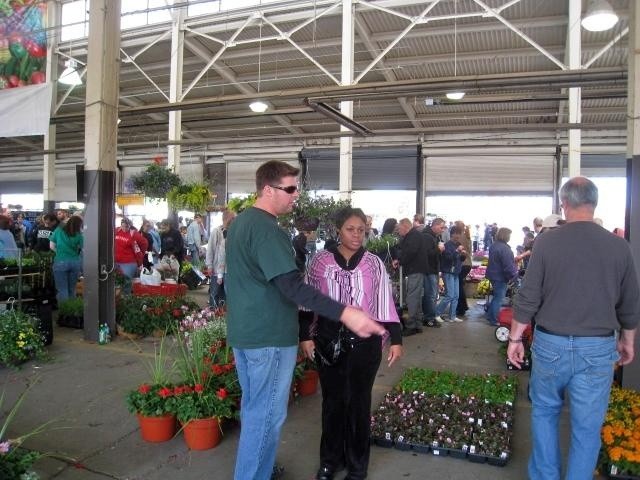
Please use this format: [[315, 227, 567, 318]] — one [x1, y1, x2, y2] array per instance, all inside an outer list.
[[371, 366, 518, 466], [119, 157, 350, 231], [117, 294, 199, 338], [290, 347, 318, 396], [1, 373, 76, 480], [56, 297, 85, 330], [179, 260, 207, 291], [469, 251, 492, 299], [1, 256, 53, 301], [1, 311, 50, 368]]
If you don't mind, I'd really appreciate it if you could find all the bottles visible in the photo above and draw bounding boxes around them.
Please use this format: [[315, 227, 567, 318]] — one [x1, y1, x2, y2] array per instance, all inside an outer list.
[[104, 323, 112, 343], [99, 324, 106, 345]]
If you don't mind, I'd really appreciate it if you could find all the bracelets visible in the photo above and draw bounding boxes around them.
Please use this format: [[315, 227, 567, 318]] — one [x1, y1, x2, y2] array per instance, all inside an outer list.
[[508, 336, 525, 344]]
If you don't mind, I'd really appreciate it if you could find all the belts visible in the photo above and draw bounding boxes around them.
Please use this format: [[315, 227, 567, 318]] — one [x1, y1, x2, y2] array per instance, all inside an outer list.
[[536, 325, 614, 337]]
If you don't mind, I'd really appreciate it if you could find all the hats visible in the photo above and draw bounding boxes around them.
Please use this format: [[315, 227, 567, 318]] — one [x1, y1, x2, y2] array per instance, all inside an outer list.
[[543, 214, 562, 228]]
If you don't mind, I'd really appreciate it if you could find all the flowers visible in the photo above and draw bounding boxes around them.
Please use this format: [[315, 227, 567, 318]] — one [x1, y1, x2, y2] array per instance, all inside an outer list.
[[179, 307, 216, 344], [200, 341, 236, 379], [0, 248, 640, 480], [140, 383, 227, 402], [601, 387, 639, 464]]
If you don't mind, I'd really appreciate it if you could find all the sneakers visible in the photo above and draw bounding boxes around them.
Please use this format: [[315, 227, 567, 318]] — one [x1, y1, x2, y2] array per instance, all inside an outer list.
[[402, 316, 463, 335]]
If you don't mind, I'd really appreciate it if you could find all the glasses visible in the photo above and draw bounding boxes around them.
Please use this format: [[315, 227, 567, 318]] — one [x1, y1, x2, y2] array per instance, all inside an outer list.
[[275, 186, 295, 193]]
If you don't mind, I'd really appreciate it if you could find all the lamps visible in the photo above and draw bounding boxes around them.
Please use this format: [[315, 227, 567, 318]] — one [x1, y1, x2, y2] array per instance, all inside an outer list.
[[445, 0, 465, 100], [58, 39, 82, 85], [581, 0, 619, 32], [248, 24, 269, 113]]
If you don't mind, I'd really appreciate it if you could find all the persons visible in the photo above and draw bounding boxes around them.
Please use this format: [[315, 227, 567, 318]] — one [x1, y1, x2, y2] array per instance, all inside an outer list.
[[502, 176, 634, 480], [300, 206, 408, 480], [224, 161, 389, 480]]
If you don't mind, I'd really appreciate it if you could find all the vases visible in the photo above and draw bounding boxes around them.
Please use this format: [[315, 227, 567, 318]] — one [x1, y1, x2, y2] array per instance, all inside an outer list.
[[176, 417, 223, 451], [136, 412, 176, 443], [599, 466, 637, 480]]
